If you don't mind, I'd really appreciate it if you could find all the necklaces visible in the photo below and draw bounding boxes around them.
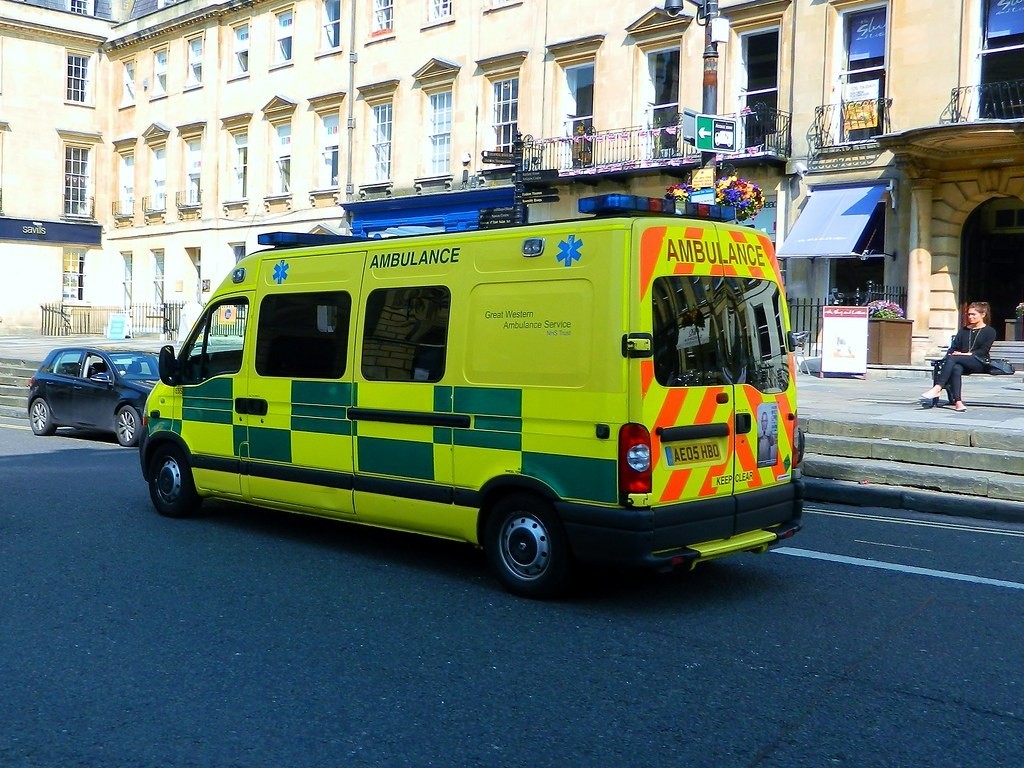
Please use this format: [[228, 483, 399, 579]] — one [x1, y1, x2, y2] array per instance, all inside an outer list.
[[969, 325, 981, 352]]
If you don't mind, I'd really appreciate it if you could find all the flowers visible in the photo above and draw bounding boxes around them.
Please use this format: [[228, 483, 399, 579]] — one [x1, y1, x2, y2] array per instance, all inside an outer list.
[[867, 300, 904, 318], [1015, 303, 1024, 319], [666, 176, 764, 220]]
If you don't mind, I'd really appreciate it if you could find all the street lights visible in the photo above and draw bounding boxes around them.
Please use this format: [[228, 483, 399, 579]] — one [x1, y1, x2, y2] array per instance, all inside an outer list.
[[664, 0, 729, 188]]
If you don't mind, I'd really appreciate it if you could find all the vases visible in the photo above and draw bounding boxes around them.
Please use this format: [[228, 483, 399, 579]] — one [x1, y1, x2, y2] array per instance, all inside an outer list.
[[867, 318, 914, 365], [1004, 319, 1024, 341]]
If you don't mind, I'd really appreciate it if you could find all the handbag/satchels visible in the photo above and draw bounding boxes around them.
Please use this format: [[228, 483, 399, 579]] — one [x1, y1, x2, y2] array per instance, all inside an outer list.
[[988, 359, 1015, 374]]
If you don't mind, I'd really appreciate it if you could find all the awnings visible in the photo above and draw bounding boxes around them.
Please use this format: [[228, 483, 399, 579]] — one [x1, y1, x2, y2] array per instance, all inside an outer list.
[[776, 178, 900, 258]]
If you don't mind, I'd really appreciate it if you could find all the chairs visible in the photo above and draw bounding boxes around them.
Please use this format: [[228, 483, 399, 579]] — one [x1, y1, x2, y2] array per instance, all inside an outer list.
[[792, 331, 810, 376], [60, 361, 142, 381]]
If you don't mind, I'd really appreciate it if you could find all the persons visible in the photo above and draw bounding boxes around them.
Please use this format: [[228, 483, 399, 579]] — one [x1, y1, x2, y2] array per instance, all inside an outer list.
[[920, 303, 995, 412]]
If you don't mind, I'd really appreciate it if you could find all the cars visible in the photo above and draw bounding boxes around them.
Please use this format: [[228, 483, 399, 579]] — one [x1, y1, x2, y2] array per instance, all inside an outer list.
[[28, 345, 160, 446]]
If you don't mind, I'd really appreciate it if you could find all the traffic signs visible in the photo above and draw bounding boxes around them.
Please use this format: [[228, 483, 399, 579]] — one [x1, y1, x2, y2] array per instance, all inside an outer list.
[[695, 114, 737, 154]]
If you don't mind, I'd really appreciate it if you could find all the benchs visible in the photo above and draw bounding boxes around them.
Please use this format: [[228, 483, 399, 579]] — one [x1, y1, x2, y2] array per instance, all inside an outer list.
[[931, 363, 1024, 408]]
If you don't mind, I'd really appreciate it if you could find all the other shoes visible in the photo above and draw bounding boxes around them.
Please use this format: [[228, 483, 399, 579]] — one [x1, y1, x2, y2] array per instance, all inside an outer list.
[[957, 407, 967, 411], [921, 393, 940, 398]]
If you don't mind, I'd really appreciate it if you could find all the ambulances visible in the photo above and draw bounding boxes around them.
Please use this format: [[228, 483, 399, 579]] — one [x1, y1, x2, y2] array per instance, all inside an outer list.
[[138, 195, 804, 597]]
